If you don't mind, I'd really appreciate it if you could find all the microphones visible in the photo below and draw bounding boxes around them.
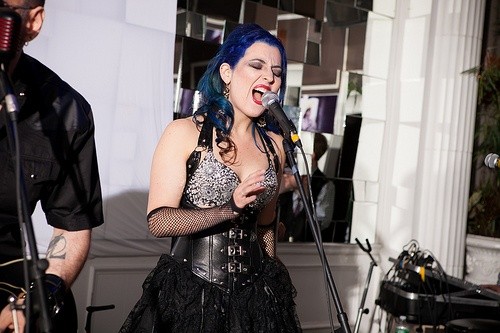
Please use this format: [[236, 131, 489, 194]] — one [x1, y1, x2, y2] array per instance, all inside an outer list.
[[0, 7, 22, 113], [485, 153, 500, 168], [261, 91, 302, 148]]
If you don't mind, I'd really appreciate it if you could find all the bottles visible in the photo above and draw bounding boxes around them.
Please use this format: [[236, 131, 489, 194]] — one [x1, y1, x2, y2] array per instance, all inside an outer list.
[[395, 315, 410, 333]]
